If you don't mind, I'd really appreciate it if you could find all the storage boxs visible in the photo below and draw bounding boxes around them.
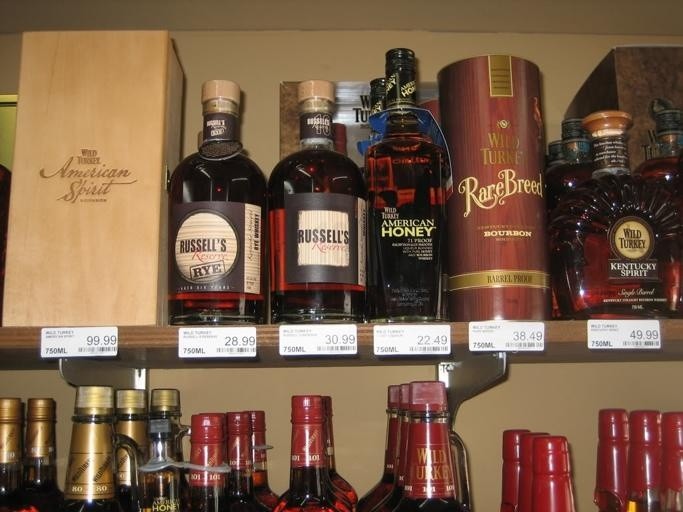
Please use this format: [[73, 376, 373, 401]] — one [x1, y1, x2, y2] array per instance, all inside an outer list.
[[564, 45, 683, 168], [1, 30, 183, 328]]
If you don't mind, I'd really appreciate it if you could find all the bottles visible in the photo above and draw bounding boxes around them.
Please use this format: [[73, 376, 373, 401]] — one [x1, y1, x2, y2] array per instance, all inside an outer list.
[[356, 382, 471, 512], [272, 395, 358, 512], [168, 82, 268, 325], [185, 410, 278, 511], [267, 80, 368, 324], [0, 398, 60, 512], [595, 407, 682, 511], [498, 429, 578, 512], [364, 47, 447, 321], [65, 383, 184, 512], [543, 107, 682, 319]]
